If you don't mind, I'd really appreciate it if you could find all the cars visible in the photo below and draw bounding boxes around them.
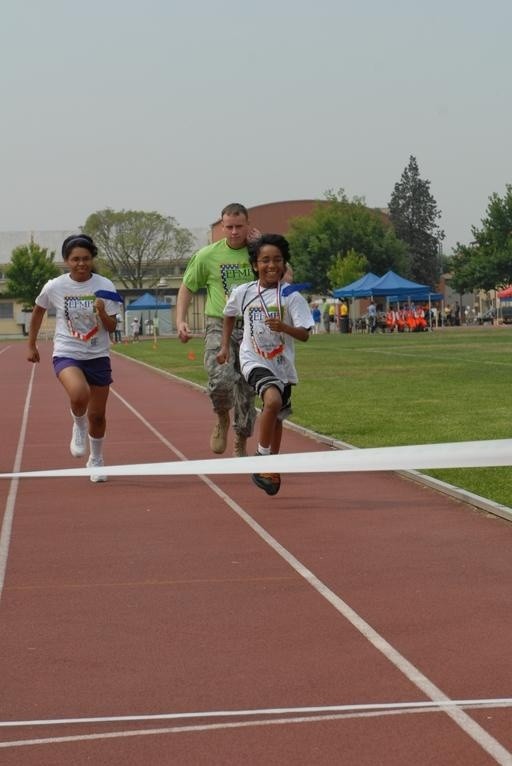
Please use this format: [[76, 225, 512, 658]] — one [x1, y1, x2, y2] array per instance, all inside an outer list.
[[477, 306, 512, 326]]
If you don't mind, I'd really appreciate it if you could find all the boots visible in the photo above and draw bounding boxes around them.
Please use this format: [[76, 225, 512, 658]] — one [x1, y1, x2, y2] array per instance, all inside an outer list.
[[210, 417, 230, 454], [231, 436, 249, 457]]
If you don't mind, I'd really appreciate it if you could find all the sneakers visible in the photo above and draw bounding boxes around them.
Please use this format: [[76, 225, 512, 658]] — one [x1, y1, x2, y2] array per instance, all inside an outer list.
[[251, 473, 281, 496], [86, 455, 107, 483], [69, 422, 88, 458]]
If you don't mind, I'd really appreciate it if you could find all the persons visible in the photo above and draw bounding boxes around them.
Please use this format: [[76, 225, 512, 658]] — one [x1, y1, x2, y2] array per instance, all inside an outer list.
[[26, 234, 122, 483], [216, 234, 316, 496], [307, 296, 460, 336], [114, 312, 123, 342], [176, 203, 293, 459], [130, 318, 141, 344]]
